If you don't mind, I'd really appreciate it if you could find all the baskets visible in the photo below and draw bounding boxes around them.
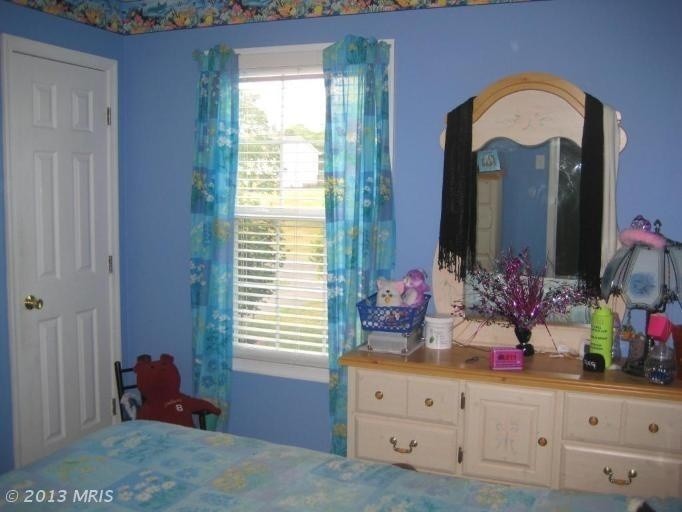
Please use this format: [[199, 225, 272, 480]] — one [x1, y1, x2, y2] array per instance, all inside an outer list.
[[356, 292, 431, 333]]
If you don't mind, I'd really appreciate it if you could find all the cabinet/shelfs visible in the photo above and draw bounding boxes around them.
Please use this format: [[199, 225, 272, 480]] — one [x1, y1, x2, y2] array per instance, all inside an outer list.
[[344, 366, 682, 500]]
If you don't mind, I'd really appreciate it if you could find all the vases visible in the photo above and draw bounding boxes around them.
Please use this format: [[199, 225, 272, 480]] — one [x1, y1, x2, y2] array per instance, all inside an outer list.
[[514, 327, 534, 356]]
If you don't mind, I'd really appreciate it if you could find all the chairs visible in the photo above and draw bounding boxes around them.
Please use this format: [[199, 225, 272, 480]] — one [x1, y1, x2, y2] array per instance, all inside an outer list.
[[114, 354, 210, 430]]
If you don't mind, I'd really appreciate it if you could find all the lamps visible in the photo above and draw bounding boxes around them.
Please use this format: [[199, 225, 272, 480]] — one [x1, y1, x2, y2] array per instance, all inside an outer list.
[[597, 220, 682, 377]]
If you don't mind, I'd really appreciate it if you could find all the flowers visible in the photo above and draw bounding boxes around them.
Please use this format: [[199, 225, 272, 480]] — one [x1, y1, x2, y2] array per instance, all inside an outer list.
[[445, 244, 591, 352]]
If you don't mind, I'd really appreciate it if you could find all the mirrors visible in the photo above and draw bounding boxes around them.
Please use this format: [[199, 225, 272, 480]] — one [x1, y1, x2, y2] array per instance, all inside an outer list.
[[439, 72, 628, 348]]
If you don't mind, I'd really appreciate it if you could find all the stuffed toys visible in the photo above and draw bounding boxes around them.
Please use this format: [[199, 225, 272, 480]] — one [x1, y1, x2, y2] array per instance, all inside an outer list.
[[132, 353, 221, 428], [399, 270, 431, 318], [375, 278, 404, 322]]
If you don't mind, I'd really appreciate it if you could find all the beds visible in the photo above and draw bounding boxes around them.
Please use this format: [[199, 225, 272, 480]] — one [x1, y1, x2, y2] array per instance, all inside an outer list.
[[0, 420, 682, 511]]
